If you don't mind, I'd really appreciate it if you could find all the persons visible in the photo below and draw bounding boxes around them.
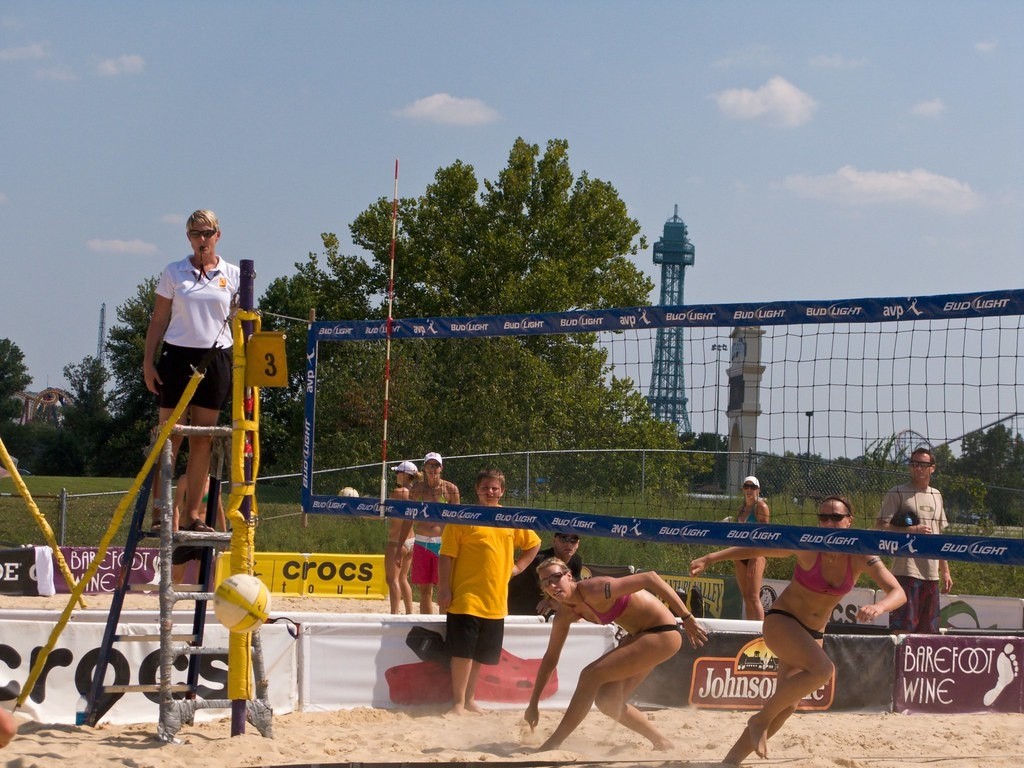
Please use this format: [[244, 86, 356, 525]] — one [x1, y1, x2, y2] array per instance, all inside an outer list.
[[141, 209, 241, 533], [437, 467, 542, 721], [729, 476, 771, 623], [0, 706, 16, 749], [508, 532, 582, 622], [690, 496, 908, 765], [523, 557, 708, 753], [172, 439, 227, 584], [360, 452, 461, 615], [877, 447, 953, 635]]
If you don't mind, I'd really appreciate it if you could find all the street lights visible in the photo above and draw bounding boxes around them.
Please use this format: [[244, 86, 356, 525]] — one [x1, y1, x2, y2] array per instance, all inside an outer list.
[[710, 344, 728, 494], [804, 411, 813, 493]]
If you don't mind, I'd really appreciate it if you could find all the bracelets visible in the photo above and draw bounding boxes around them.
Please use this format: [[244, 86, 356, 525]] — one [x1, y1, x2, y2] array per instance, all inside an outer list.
[[681, 613, 690, 621]]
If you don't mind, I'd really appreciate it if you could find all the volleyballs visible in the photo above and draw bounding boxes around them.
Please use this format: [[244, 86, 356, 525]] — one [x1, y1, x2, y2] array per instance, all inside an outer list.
[[338, 486, 358, 498], [214, 573, 274, 633]]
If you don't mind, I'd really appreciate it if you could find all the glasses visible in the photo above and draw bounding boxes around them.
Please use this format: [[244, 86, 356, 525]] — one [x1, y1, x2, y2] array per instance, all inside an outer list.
[[539, 572, 567, 587], [189, 228, 218, 239], [560, 536, 579, 545], [909, 460, 933, 469], [743, 483, 758, 490], [818, 514, 851, 522], [428, 463, 440, 468], [395, 471, 404, 474]]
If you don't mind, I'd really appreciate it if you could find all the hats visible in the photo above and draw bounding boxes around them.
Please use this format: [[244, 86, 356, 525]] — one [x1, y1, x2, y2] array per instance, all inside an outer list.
[[423, 452, 442, 466], [391, 462, 418, 478], [890, 511, 919, 527], [744, 476, 760, 487]]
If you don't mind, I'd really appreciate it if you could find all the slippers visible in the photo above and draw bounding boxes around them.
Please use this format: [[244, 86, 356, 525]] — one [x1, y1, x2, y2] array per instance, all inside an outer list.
[[151, 519, 214, 532]]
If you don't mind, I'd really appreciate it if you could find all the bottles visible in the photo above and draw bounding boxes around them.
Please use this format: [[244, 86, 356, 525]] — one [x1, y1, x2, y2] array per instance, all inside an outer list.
[[76, 693, 88, 725]]
